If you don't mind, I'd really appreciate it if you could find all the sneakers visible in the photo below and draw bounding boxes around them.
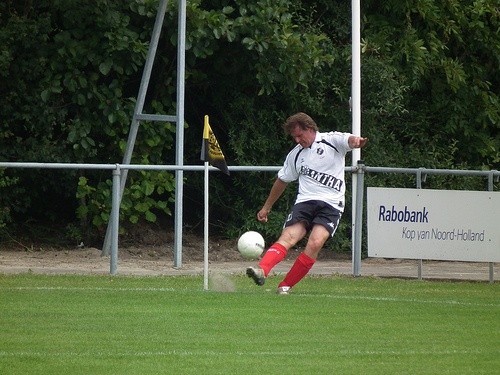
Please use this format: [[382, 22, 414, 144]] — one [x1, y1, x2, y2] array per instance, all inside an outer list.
[[278, 286, 291, 295], [246, 266, 265, 286]]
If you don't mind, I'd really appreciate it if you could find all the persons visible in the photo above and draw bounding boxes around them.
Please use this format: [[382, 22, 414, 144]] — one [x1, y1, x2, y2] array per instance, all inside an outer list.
[[246, 113, 367, 295]]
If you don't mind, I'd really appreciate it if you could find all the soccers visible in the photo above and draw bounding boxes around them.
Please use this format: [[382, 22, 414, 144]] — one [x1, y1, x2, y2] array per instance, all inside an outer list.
[[237, 230, 266, 259]]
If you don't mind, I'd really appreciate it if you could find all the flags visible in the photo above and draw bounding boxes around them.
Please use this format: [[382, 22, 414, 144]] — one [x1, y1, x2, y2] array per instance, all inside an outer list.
[[201, 115, 230, 176]]
